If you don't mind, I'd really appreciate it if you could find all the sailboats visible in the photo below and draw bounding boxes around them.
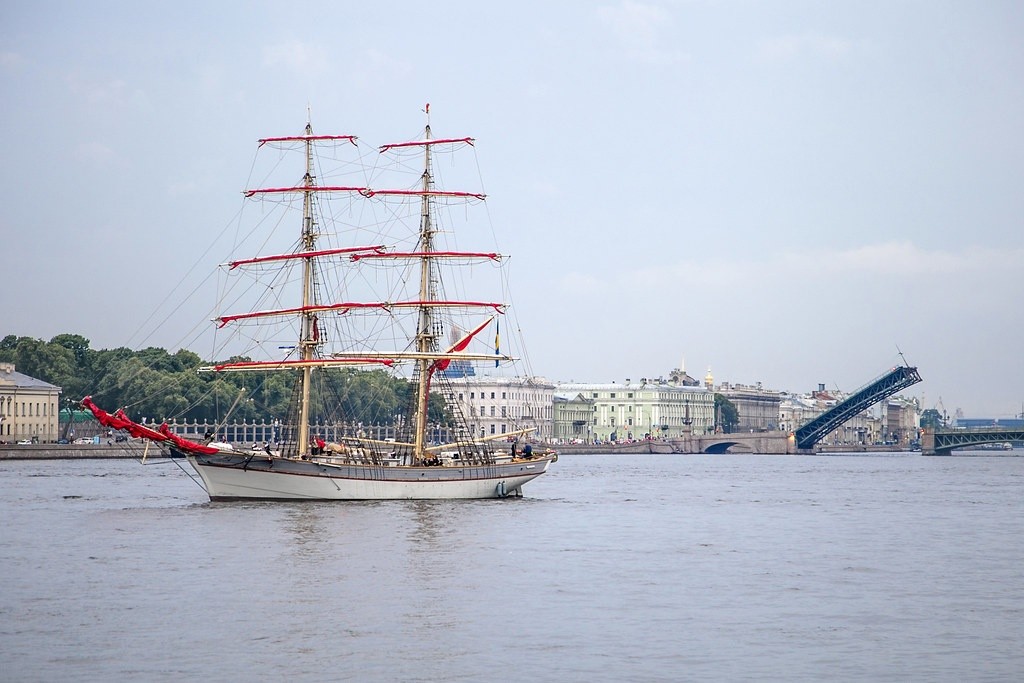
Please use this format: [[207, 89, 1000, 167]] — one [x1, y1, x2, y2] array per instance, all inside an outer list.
[[61, 98, 559, 503]]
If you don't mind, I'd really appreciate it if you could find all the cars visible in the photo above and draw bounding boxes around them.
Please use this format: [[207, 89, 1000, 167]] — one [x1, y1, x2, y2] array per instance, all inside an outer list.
[[108, 434, 128, 446], [59, 439, 69, 444], [17, 439, 31, 445], [71, 436, 95, 445]]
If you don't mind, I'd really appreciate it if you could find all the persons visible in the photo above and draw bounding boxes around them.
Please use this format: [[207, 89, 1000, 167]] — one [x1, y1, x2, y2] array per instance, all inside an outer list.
[[595, 434, 659, 446], [204, 427, 326, 455], [414, 439, 532, 467]]
